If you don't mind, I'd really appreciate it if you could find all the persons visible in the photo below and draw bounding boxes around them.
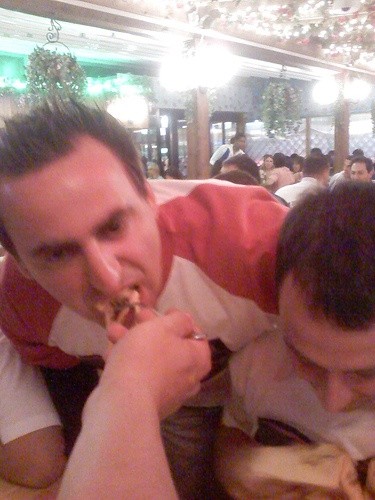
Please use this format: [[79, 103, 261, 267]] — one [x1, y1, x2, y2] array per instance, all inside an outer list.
[[147, 161, 163, 179], [0, 94, 290, 500], [0, 330, 64, 488], [220, 156, 288, 207], [210, 134, 246, 177], [259, 148, 326, 193], [214, 181, 375, 500], [329, 149, 375, 192], [165, 167, 183, 179], [275, 156, 330, 208], [56, 306, 214, 500]]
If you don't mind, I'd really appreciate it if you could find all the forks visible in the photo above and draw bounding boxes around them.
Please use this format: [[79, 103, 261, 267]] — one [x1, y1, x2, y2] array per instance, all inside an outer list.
[[108, 296, 208, 340]]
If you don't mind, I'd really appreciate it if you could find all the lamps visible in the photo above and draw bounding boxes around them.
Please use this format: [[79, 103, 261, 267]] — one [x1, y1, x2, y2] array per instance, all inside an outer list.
[[311, 68, 372, 106], [151, 28, 241, 97]]
